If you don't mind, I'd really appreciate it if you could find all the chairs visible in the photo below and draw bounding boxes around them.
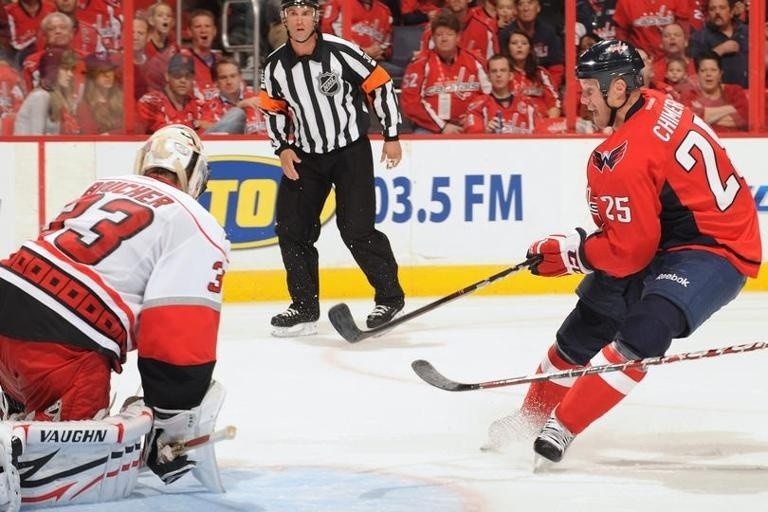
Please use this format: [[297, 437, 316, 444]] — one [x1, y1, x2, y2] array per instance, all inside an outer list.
[[390, 25, 425, 88]]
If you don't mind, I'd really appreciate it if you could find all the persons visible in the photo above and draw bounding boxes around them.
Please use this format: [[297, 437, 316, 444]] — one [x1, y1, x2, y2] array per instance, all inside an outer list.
[[262, 0, 405, 330], [2, 0, 748, 136], [487, 36, 762, 463], [1, 124, 235, 512]]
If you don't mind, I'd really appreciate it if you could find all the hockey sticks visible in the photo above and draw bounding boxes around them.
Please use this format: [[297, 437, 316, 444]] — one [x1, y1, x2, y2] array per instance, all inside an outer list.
[[328, 228, 587, 342], [412, 341, 767, 392]]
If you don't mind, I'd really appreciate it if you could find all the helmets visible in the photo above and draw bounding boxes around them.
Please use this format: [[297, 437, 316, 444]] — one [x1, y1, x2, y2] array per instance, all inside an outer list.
[[576, 39, 646, 79], [134, 125, 210, 201], [280, 0, 320, 12]]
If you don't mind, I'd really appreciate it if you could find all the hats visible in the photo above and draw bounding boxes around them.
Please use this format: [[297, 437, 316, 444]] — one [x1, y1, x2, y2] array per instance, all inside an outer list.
[[78, 51, 120, 75], [169, 55, 194, 74]]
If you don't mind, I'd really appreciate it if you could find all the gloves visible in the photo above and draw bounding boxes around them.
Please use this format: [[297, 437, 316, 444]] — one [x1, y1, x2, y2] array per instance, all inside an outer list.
[[142, 409, 198, 485], [526, 227, 592, 277]]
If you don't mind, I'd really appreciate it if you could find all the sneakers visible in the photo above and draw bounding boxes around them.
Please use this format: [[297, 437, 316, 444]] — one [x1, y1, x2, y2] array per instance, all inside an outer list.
[[488, 411, 534, 441], [271, 303, 320, 327], [534, 413, 574, 462], [367, 297, 405, 328]]
